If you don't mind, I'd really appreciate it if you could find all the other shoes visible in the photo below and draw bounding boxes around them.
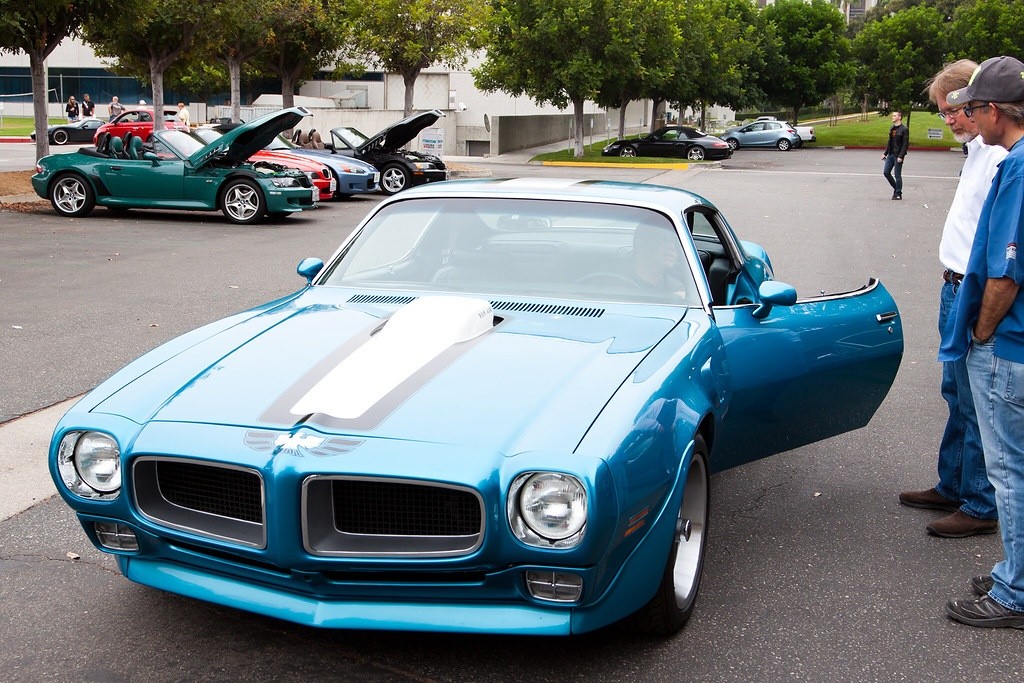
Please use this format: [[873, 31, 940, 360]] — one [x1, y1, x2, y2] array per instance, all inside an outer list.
[[899, 487, 959, 511], [926, 508, 997, 537], [892, 191, 901, 200]]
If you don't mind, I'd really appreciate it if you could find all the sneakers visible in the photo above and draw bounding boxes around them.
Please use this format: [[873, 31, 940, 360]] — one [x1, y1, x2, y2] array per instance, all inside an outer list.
[[971, 573, 995, 596], [945, 595, 1024, 630]]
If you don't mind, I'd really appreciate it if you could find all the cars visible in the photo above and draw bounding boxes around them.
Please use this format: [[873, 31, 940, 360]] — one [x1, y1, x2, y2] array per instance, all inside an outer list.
[[720, 121, 800, 151], [92, 108, 189, 148]]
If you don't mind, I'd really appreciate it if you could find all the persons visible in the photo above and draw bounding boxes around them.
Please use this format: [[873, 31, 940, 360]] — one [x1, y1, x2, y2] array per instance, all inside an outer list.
[[65, 96, 80, 124], [882, 110, 909, 200], [138, 100, 146, 108], [935, 55, 1024, 629], [898, 59, 998, 539], [177, 103, 190, 127], [108, 96, 127, 124], [80, 94, 96, 120]]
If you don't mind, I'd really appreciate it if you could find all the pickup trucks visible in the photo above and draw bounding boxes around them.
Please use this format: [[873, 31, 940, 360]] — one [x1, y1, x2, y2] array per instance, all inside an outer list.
[[751, 117, 817, 148]]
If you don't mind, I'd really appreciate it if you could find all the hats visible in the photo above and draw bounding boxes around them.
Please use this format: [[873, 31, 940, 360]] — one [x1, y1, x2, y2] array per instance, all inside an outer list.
[[946, 55, 1024, 105], [140, 100, 146, 104]]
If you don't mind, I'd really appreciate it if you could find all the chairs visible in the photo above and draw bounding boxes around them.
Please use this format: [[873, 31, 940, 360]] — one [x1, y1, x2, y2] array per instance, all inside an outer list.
[[97, 132, 145, 160], [292, 129, 325, 150]]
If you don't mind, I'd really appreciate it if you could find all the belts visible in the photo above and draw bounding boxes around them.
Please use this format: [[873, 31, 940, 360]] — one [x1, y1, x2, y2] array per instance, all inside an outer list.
[[943, 270, 964, 285]]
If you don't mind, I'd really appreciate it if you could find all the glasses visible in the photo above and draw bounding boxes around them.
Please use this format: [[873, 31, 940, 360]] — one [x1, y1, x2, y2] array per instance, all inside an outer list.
[[938, 104, 965, 120], [963, 105, 990, 118]]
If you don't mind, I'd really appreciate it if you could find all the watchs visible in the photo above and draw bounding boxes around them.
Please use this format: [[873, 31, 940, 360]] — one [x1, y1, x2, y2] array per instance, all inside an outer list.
[[971, 324, 988, 345]]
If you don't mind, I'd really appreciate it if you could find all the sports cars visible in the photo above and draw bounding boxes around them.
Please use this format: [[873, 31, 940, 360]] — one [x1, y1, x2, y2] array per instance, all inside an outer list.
[[30, 118, 109, 145], [602, 125, 734, 162], [286, 109, 450, 196], [155, 127, 339, 202], [29, 106, 320, 225], [48, 177, 906, 641], [213, 124, 381, 199]]
